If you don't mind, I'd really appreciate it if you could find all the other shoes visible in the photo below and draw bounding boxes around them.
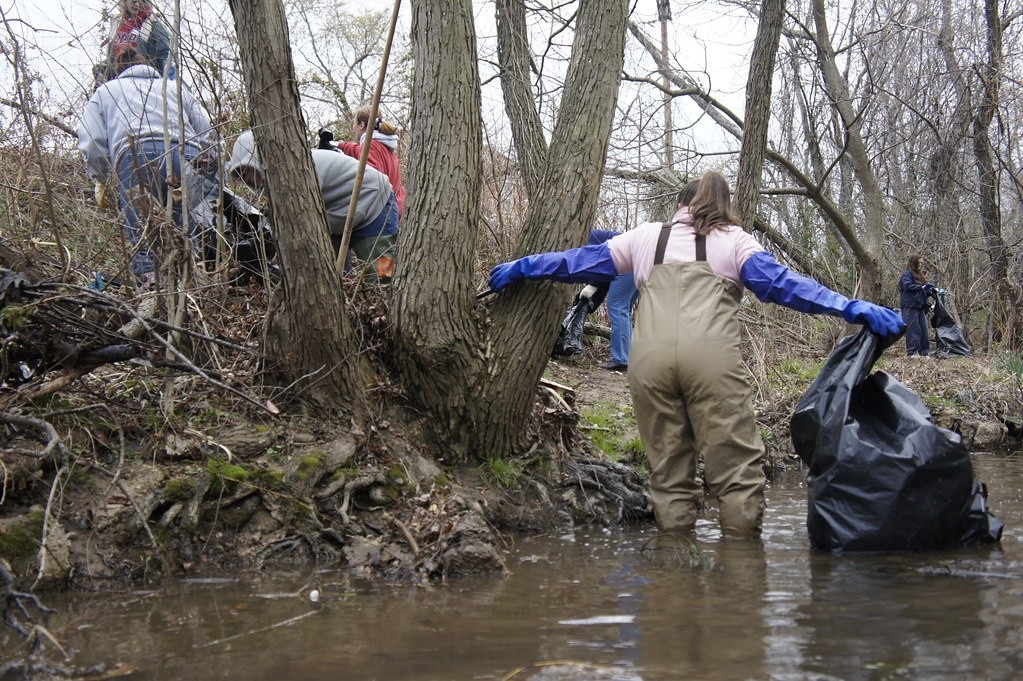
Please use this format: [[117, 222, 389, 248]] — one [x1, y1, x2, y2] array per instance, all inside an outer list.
[[136, 270, 162, 291], [596, 359, 628, 371]]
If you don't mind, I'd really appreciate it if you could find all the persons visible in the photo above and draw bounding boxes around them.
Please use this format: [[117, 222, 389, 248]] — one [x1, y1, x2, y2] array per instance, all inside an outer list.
[[329, 105, 406, 220], [579, 229, 642, 370], [109, 0, 176, 82], [78, 44, 215, 287], [230, 130, 399, 301], [899, 254, 933, 356], [487, 173, 907, 539]]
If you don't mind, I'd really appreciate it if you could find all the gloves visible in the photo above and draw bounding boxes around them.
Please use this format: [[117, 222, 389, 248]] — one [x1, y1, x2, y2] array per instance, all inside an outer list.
[[93, 64, 106, 80], [739, 252, 905, 337], [95, 181, 111, 208], [487, 240, 619, 294], [579, 284, 597, 300], [164, 63, 175, 80], [922, 283, 934, 290]]
